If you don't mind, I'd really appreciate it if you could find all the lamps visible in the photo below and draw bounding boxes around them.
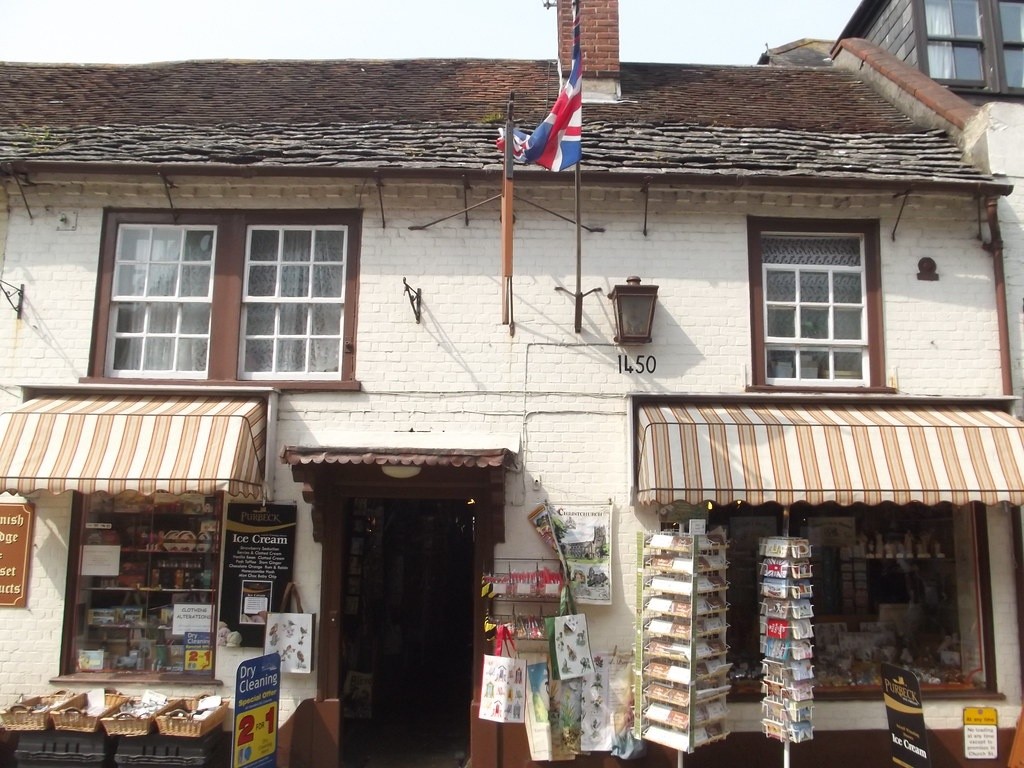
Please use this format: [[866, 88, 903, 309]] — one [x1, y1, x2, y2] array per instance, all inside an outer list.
[[605, 274, 660, 342]]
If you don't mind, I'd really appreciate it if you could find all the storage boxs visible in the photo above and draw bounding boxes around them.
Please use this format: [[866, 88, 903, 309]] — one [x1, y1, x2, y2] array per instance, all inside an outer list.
[[345, 687, 385, 720]]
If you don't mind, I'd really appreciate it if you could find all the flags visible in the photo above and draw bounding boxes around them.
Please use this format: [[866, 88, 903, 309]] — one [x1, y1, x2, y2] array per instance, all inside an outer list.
[[497, 5, 582, 173]]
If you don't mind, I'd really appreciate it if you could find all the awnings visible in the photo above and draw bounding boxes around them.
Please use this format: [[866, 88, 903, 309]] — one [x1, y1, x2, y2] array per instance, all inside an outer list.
[[0, 395, 266, 500], [637, 402, 1024, 508]]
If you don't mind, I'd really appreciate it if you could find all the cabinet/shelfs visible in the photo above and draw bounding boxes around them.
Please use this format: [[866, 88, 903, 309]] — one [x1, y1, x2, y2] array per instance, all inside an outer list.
[[75, 508, 220, 676]]
